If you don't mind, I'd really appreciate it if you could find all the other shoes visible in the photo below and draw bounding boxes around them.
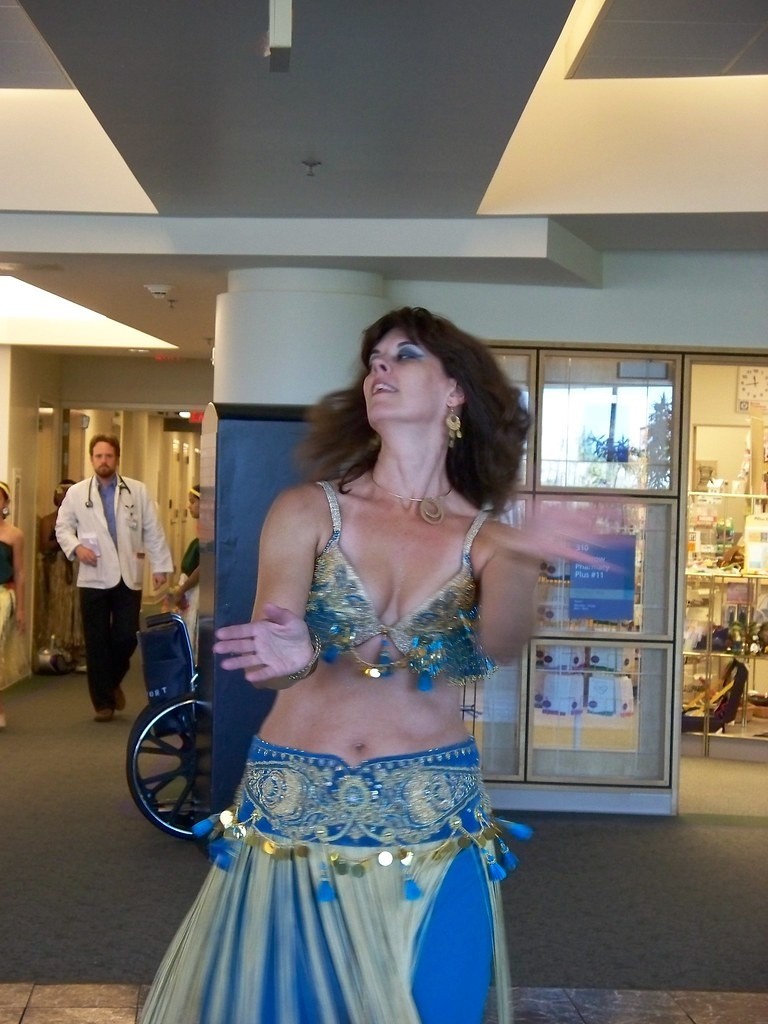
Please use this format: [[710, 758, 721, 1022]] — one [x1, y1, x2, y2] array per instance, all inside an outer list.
[[94, 709, 114, 722], [0, 712, 6, 727], [113, 685, 125, 711], [75, 656, 87, 672]]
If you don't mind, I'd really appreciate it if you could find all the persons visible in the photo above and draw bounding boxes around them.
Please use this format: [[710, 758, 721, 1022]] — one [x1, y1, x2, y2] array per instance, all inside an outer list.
[[139, 305, 635, 1024], [0, 431, 199, 732]]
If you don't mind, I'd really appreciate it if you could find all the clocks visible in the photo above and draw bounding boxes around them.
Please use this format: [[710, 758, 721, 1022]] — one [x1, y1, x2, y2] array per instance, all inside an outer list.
[[736, 365, 768, 402]]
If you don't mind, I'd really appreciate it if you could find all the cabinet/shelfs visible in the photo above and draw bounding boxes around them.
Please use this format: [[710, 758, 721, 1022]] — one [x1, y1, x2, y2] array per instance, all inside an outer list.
[[680, 570, 768, 759]]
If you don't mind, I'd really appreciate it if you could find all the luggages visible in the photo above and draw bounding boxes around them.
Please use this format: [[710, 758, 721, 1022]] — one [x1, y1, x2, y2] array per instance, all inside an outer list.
[[681, 660, 748, 733]]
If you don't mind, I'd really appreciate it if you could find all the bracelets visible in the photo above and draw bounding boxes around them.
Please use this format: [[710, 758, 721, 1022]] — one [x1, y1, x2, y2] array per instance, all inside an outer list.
[[290, 629, 324, 679], [175, 586, 182, 596]]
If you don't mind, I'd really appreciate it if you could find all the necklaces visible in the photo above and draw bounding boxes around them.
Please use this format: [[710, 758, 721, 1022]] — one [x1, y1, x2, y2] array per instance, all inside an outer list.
[[367, 468, 454, 524]]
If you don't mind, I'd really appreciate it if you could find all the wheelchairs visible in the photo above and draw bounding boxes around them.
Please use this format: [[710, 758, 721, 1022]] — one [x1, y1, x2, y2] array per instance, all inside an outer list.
[[125, 612, 213, 841]]
